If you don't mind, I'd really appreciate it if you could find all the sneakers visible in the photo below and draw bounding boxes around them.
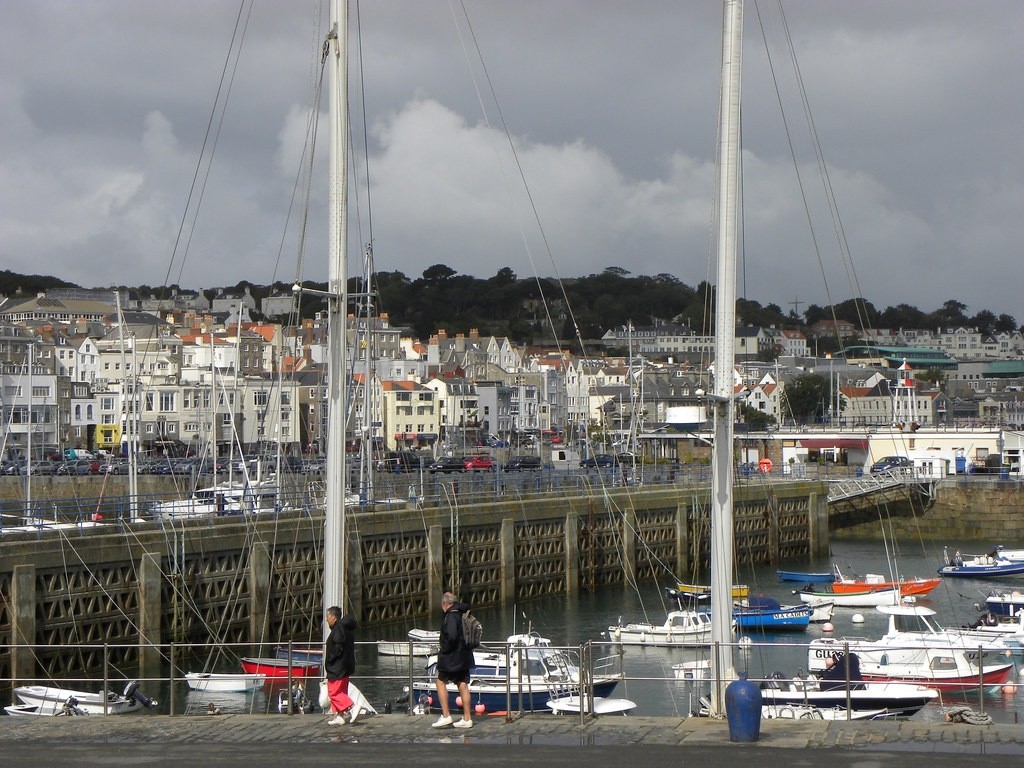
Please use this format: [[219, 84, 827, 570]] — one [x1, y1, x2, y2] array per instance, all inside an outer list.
[[432, 714, 453, 727], [328, 716, 346, 725], [452, 717, 473, 728], [350, 705, 362, 723]]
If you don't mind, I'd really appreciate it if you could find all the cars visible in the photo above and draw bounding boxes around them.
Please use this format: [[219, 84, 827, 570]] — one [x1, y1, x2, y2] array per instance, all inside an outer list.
[[869, 455, 910, 478], [98, 457, 133, 475], [428, 456, 467, 475], [579, 453, 615, 469], [19, 460, 55, 477], [500, 455, 541, 473], [463, 455, 494, 473], [738, 462, 758, 477], [56, 459, 92, 475], [615, 450, 641, 464]]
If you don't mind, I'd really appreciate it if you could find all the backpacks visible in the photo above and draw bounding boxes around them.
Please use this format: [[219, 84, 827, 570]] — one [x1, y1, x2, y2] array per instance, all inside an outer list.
[[450, 610, 482, 649]]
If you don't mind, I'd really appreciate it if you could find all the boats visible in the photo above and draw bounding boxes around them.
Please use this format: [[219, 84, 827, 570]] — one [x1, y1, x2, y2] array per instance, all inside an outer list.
[[606, 606, 737, 649], [0, 1, 1024, 724], [375, 639, 441, 657], [184, 671, 267, 694], [13, 680, 159, 716], [407, 628, 441, 642], [3, 695, 91, 717], [272, 645, 360, 666], [671, 658, 711, 685], [238, 656, 322, 682], [686, 653, 940, 719]]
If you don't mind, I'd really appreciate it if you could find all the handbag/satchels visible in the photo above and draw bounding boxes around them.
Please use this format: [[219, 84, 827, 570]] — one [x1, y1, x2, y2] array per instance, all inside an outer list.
[[319, 680, 331, 708]]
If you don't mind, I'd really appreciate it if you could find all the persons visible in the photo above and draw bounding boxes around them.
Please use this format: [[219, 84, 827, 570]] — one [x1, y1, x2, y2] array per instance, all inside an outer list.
[[324, 606, 362, 727], [431, 592, 476, 728]]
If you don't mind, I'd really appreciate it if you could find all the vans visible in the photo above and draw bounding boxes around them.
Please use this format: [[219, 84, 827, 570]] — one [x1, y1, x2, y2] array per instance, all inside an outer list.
[[384, 451, 422, 474]]
